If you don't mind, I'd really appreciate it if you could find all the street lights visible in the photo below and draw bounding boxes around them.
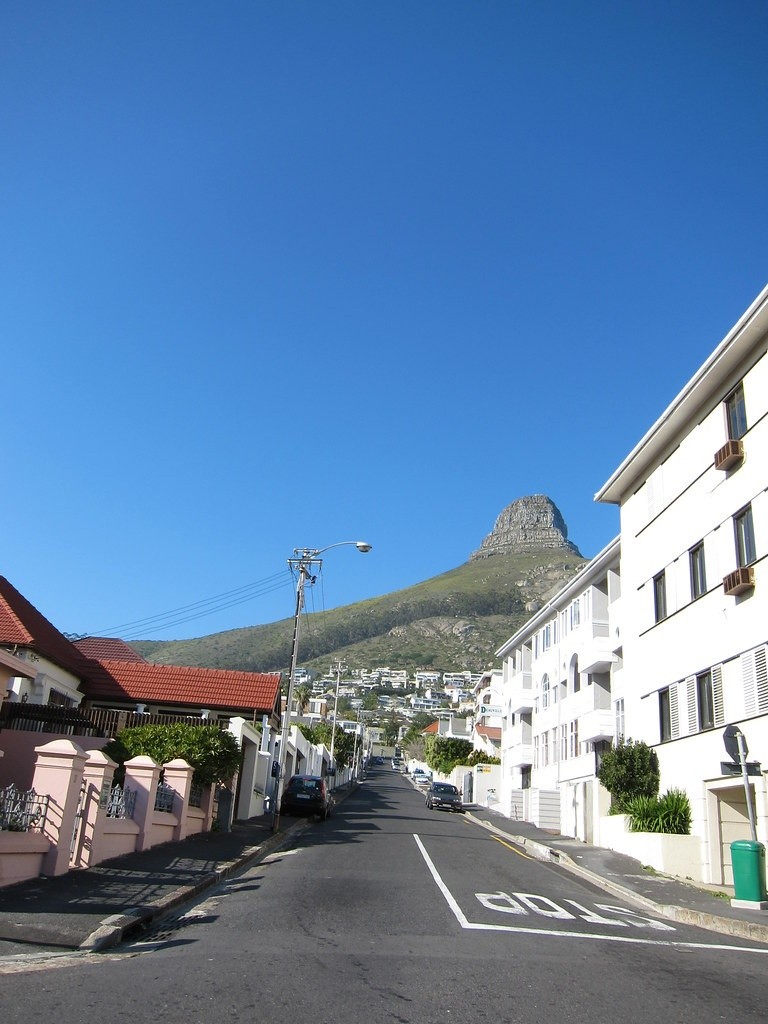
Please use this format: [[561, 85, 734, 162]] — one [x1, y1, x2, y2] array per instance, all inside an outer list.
[[272, 538, 369, 834]]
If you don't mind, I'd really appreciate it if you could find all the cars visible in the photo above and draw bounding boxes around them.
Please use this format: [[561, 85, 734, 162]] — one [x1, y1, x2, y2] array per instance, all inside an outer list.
[[410, 768, 428, 784], [391, 757, 404, 769], [426, 782, 462, 812], [280, 774, 330, 821], [377, 758, 383, 765]]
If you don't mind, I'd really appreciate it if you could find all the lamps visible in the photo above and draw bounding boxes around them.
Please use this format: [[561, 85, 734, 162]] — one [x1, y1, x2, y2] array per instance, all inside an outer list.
[[22, 693, 28, 703]]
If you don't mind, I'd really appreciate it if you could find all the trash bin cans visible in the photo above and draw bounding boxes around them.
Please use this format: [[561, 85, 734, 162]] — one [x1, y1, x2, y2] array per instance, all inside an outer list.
[[730, 839, 768, 902]]
